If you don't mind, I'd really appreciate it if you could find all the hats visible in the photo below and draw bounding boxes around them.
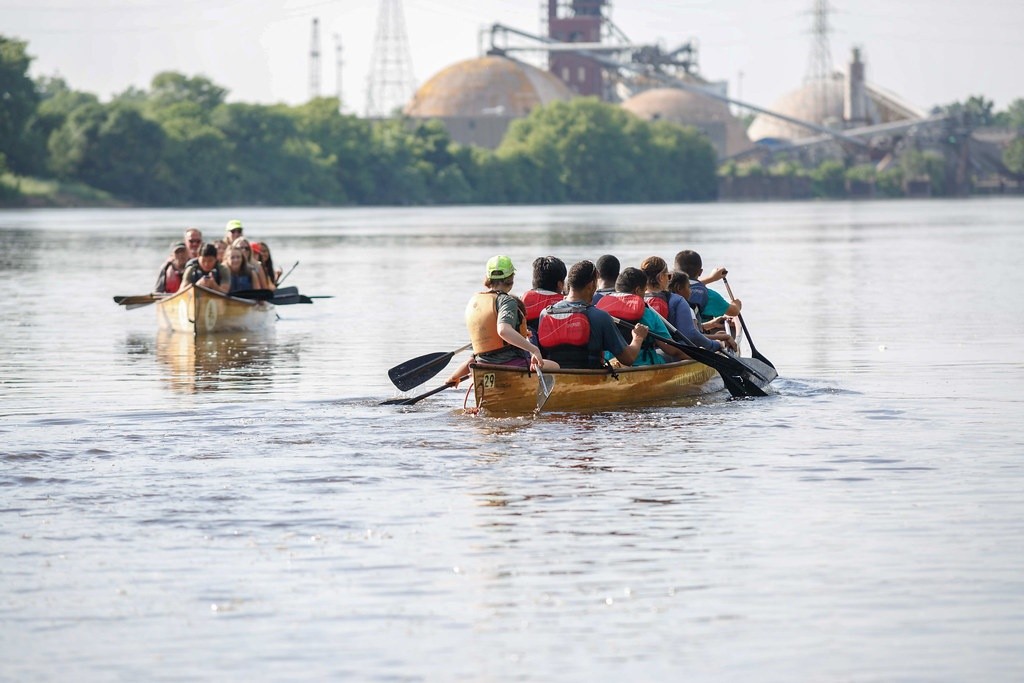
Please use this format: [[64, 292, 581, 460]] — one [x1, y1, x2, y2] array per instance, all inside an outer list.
[[249, 242, 260, 252], [226, 220, 243, 232], [486, 255, 516, 279], [173, 241, 186, 253]]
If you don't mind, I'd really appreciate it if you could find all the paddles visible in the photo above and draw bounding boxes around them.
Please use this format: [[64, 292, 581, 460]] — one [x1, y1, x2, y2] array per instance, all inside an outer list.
[[309, 295, 335, 299], [722, 270, 780, 377], [611, 315, 745, 376], [721, 316, 738, 356], [379, 373, 471, 405], [276, 260, 300, 287], [297, 295, 313, 304], [113, 286, 302, 311], [644, 301, 769, 397], [387, 342, 472, 392], [527, 328, 556, 410], [718, 348, 777, 389]]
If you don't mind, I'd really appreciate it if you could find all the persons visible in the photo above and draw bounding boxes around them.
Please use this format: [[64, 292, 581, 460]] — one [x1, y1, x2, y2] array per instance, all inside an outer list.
[[538, 260, 648, 369], [594, 267, 692, 368], [156, 219, 283, 293], [445, 250, 742, 390], [467, 255, 560, 372]]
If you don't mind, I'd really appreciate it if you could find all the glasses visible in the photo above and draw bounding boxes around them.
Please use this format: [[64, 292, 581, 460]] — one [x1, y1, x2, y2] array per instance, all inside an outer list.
[[230, 230, 241, 233], [660, 273, 672, 280]]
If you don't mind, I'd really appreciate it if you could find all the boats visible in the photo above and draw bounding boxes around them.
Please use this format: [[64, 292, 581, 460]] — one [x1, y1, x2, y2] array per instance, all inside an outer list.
[[468, 314, 743, 418], [157, 283, 266, 334]]
